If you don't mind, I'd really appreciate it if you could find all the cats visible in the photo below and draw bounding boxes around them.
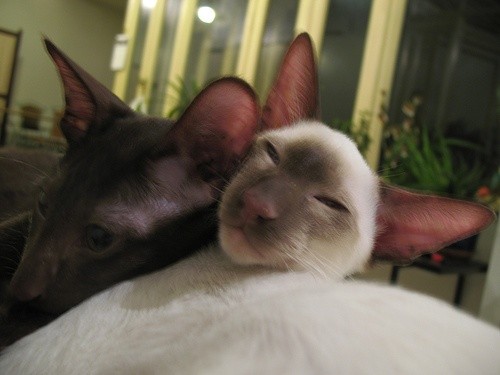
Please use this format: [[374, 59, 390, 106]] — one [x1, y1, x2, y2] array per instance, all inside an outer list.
[[1, 30, 500, 375]]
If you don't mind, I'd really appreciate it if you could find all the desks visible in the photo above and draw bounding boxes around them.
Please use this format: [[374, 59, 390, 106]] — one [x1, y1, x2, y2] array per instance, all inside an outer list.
[[5, 122, 70, 154], [388, 249, 488, 306]]
